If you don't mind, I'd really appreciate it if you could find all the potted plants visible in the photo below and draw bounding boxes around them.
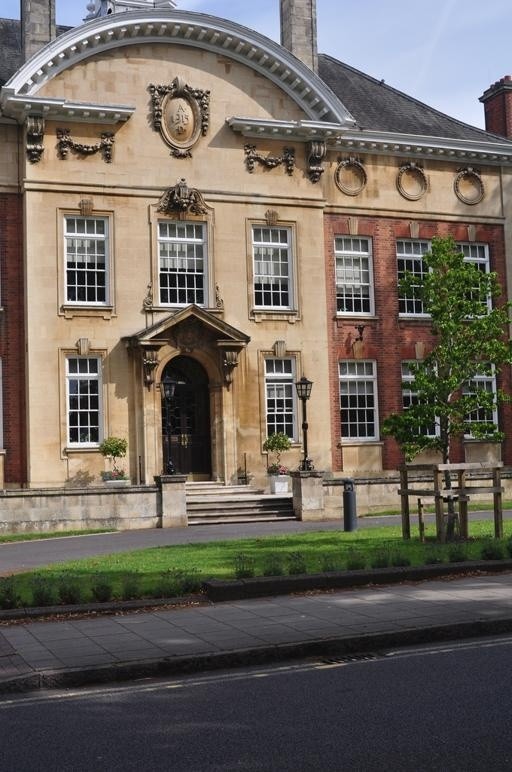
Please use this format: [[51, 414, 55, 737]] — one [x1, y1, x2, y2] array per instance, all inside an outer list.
[[262, 431, 292, 495], [98, 437, 132, 485]]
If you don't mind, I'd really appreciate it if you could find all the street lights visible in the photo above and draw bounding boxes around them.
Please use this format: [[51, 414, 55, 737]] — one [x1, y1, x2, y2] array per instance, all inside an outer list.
[[294, 375, 315, 471]]
